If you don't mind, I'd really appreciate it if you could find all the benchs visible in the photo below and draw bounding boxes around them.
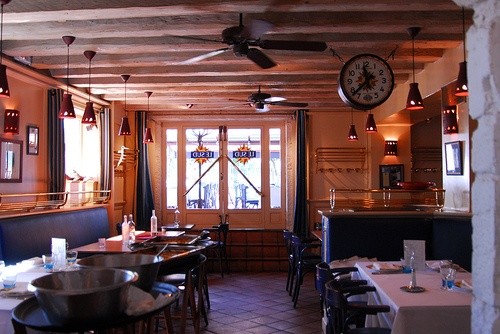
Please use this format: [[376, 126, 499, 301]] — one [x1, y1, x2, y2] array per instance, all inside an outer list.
[[0, 208, 109, 267]]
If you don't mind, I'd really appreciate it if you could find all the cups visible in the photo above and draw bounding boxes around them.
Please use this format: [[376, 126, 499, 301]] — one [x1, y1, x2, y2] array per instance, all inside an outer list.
[[67, 251, 77, 266], [441, 268, 456, 290], [42, 254, 53, 271], [401, 258, 415, 273], [3, 274, 17, 289], [98, 238, 105, 247]]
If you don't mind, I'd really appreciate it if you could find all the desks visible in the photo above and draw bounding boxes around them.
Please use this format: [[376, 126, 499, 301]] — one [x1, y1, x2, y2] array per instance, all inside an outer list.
[[162, 223, 195, 230], [0, 230, 198, 334], [108, 231, 195, 245], [348, 261, 471, 334], [71, 241, 140, 254], [312, 231, 322, 242]]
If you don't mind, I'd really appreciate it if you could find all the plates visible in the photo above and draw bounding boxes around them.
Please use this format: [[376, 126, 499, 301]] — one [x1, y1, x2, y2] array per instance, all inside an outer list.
[[396, 182, 437, 190], [400, 286, 424, 293]]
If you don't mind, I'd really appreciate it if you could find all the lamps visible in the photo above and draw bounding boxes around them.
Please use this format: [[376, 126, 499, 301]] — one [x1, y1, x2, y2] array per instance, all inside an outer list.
[[118, 75, 131, 135], [0, 0, 12, 98], [5, 108, 20, 135], [142, 92, 153, 142], [81, 51, 96, 124], [58, 37, 76, 118], [406, 27, 425, 110], [384, 140, 398, 156], [442, 105, 459, 135], [347, 108, 359, 141], [365, 109, 377, 133], [454, 7, 469, 97]]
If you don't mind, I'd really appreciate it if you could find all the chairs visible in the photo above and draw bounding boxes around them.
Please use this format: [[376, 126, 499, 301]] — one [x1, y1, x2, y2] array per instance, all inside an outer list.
[[127, 213, 229, 334], [282, 228, 392, 334]]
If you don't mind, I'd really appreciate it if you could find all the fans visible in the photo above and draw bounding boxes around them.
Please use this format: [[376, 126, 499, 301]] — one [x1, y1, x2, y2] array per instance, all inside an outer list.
[[210, 85, 308, 110], [164, 14, 327, 70]]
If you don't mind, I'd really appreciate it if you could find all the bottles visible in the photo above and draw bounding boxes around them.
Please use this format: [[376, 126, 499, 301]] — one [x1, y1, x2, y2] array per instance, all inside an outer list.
[[151, 210, 157, 235], [122, 214, 136, 245]]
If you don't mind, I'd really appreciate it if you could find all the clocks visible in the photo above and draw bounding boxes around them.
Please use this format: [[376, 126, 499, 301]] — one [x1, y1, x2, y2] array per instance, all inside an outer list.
[[337, 53, 395, 111]]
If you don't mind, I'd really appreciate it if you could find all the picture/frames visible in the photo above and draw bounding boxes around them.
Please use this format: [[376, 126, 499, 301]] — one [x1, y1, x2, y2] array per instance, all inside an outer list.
[[379, 164, 404, 189], [0, 138, 23, 184], [444, 140, 462, 176], [26, 126, 40, 156]]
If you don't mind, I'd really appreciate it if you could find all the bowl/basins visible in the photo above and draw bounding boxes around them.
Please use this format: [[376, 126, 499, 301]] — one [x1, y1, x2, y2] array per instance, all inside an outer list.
[[29, 266, 140, 325]]
